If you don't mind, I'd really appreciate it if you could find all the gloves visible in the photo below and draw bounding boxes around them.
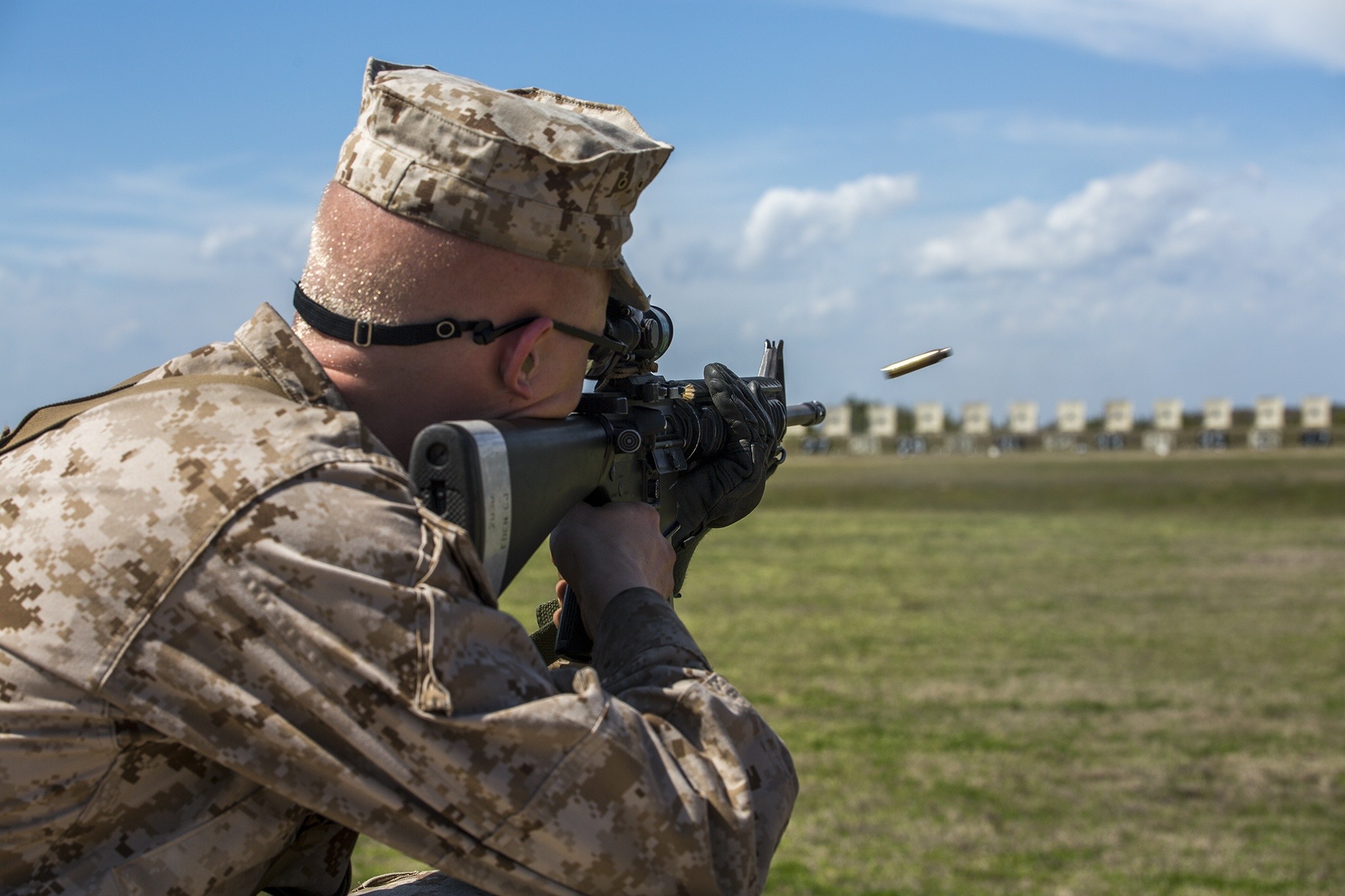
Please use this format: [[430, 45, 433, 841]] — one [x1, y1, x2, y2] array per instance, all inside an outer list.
[[670, 364, 779, 597]]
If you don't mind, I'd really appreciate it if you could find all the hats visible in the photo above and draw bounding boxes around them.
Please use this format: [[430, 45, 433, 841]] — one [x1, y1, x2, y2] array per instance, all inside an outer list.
[[331, 57, 675, 311]]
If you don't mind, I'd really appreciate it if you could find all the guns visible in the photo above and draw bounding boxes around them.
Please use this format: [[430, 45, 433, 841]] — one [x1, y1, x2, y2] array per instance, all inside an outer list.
[[406, 296, 827, 665]]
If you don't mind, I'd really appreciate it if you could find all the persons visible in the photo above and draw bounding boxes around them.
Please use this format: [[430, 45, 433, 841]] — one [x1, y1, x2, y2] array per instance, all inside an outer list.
[[0, 57, 799, 896]]
